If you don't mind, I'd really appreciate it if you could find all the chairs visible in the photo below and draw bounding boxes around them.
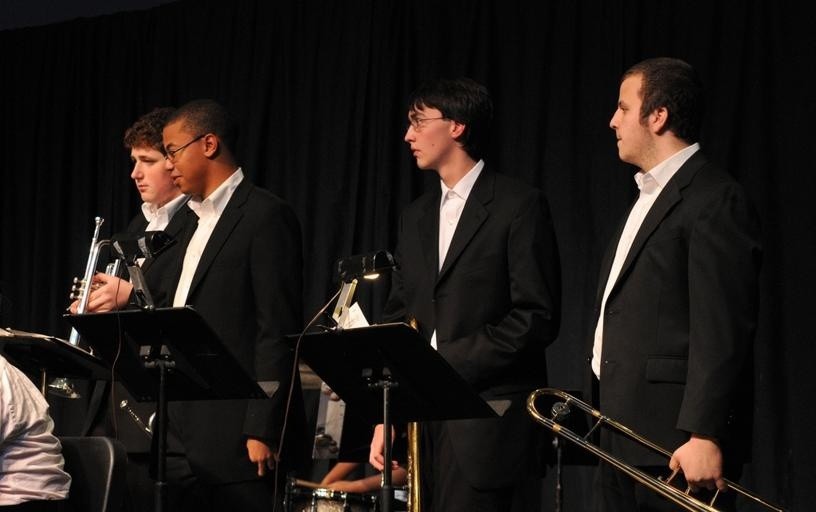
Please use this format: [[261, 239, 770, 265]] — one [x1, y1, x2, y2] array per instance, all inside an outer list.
[[55, 433, 133, 512]]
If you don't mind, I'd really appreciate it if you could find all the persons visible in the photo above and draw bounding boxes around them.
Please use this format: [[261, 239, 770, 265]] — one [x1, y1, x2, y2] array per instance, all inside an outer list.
[[64, 105, 200, 511], [128, 99, 310, 512], [370, 76, 563, 512], [0, 349, 73, 511], [319, 406, 408, 494], [583, 57, 764, 510]]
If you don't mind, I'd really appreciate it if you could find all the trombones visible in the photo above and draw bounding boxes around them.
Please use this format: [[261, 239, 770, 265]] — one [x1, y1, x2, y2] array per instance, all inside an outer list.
[[528, 389, 785, 512]]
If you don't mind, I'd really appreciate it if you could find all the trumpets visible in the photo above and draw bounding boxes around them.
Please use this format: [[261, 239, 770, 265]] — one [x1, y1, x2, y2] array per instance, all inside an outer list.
[[48, 216, 122, 398]]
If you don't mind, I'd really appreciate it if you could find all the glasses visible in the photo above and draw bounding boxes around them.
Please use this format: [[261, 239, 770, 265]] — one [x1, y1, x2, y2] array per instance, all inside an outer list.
[[410, 114, 444, 128], [166, 133, 206, 159]]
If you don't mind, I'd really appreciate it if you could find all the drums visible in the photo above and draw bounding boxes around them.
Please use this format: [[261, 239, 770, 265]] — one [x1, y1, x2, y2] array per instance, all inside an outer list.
[[301, 488, 378, 512]]
